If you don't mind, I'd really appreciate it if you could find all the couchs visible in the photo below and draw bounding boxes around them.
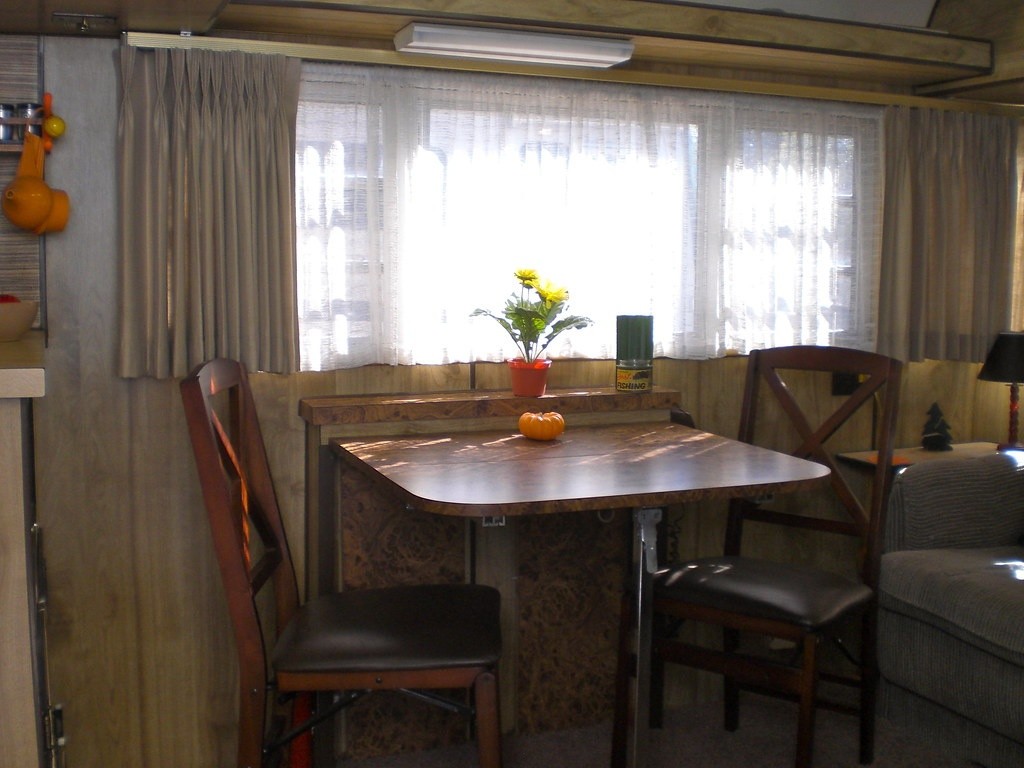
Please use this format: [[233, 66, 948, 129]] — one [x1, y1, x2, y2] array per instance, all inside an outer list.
[[876, 450, 1024, 768]]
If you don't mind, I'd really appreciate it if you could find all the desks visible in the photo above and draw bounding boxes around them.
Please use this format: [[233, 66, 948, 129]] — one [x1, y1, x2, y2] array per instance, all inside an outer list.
[[327, 421, 833, 768], [833, 442, 999, 563]]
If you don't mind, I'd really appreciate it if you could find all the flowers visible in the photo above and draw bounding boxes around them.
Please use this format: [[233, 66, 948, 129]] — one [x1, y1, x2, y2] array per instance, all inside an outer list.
[[468, 268, 595, 363]]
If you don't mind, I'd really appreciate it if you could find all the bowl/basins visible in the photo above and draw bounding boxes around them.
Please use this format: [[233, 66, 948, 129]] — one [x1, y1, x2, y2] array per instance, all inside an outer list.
[[0, 299, 39, 342]]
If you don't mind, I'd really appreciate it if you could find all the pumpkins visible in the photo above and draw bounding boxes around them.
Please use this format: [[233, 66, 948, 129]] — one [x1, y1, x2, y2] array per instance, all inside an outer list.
[[519, 411, 564, 440], [726, 349, 737, 356]]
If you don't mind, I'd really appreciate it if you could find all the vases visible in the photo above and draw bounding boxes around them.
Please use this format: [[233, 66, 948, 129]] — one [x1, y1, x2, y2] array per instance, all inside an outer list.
[[504, 358, 554, 398]]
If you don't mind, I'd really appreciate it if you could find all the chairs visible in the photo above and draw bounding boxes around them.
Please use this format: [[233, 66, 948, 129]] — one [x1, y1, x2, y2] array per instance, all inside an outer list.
[[648, 345, 905, 768], [179, 355, 506, 768]]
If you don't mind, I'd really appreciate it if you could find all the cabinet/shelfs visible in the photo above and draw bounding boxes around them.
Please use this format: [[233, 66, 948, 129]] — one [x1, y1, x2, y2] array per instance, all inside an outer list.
[[0, 369, 68, 768]]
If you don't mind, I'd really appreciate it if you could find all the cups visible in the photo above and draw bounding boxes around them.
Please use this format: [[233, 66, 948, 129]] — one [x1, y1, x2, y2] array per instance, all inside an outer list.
[[0, 103, 16, 144], [16, 102, 43, 145]]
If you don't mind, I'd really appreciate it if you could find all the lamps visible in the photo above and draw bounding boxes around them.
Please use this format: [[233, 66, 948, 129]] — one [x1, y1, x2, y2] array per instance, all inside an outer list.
[[976, 331, 1024, 452], [391, 20, 635, 72]]
